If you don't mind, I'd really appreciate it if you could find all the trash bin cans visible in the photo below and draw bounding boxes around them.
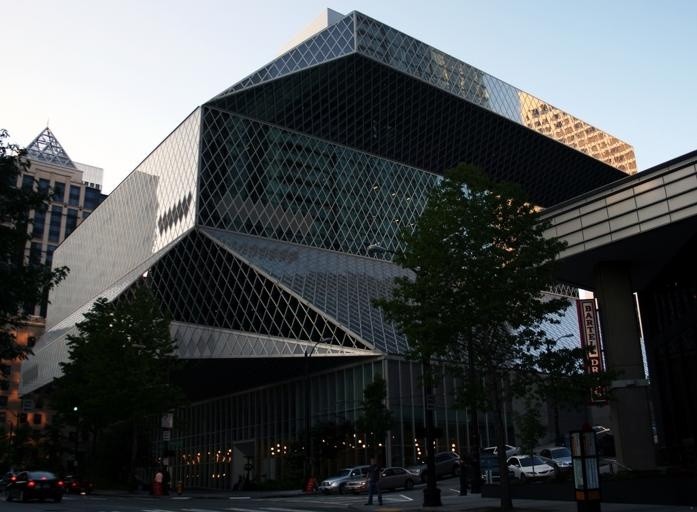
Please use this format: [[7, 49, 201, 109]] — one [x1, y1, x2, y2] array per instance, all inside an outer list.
[[152, 480, 162, 497]]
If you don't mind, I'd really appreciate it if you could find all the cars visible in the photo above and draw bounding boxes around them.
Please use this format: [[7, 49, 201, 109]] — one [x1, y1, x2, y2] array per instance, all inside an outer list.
[[1, 471, 94, 502], [319, 425, 612, 492]]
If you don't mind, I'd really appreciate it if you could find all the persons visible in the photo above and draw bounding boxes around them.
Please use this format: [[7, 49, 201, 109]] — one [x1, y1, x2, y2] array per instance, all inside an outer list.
[[364, 458, 383, 506]]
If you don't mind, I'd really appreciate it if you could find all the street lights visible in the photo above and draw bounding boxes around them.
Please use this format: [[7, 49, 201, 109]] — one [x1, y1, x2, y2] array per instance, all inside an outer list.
[[305, 338, 333, 492], [367, 244, 441, 507]]
